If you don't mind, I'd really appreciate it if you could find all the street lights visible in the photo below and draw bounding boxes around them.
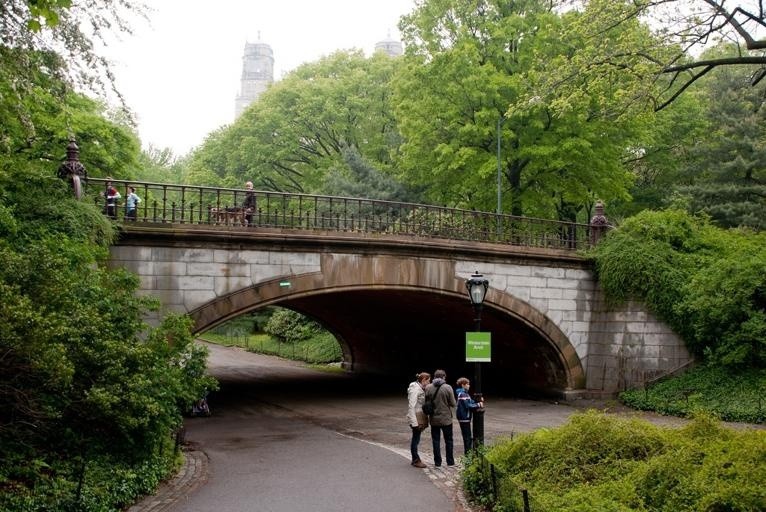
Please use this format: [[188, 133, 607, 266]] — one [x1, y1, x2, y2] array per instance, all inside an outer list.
[[463, 270, 492, 451]]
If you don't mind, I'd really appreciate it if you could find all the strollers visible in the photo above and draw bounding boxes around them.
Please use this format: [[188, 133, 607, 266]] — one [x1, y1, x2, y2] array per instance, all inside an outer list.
[[211, 206, 249, 228]]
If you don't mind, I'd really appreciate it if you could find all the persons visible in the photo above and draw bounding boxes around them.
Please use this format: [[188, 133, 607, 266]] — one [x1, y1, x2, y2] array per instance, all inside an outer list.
[[94, 191, 104, 205], [102, 177, 122, 220], [123, 185, 141, 221], [455, 377, 487, 455], [424, 369, 457, 467], [240, 180, 257, 227], [405, 372, 431, 469]]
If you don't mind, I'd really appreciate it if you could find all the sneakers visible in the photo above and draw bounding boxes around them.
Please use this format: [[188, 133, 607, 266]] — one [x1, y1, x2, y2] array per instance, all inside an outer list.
[[411, 460, 426, 468], [448, 463, 454, 466], [435, 462, 441, 466]]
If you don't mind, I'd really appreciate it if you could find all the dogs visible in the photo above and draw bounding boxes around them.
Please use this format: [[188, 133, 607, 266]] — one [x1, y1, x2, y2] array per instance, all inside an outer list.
[[211, 204, 256, 227]]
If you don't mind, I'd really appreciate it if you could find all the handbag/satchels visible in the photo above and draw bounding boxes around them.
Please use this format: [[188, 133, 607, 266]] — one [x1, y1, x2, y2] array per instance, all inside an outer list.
[[422, 404, 434, 415]]
